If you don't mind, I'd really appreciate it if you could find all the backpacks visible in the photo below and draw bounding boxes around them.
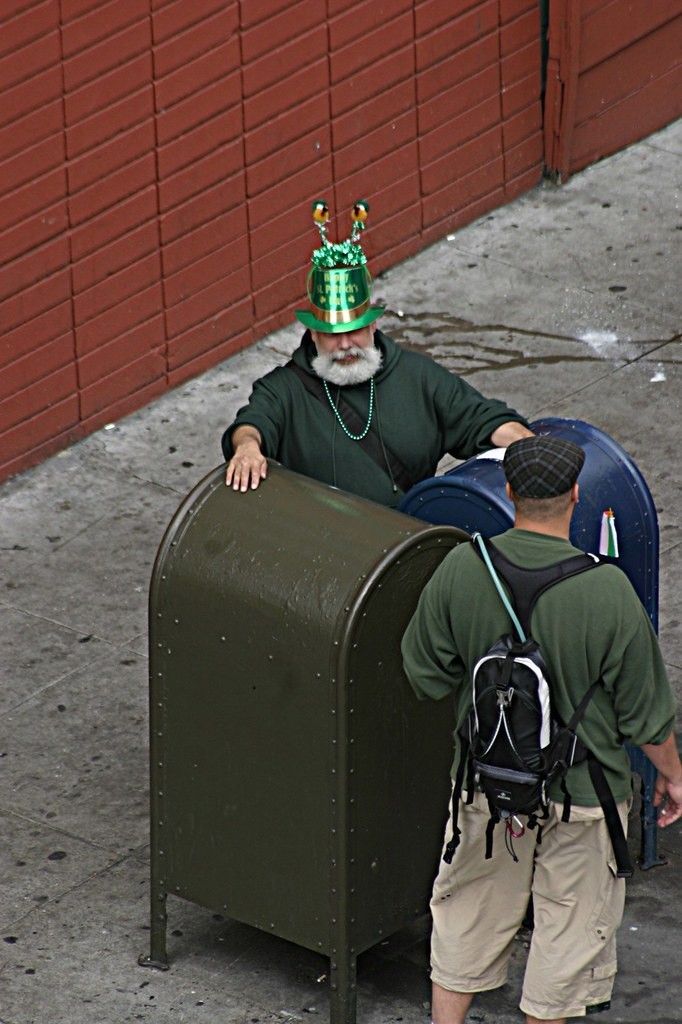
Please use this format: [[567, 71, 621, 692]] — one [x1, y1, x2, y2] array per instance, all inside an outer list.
[[470, 533, 610, 814]]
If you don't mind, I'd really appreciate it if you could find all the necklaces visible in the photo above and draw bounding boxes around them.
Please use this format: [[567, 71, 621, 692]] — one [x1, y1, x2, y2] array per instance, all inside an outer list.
[[324, 376, 375, 440]]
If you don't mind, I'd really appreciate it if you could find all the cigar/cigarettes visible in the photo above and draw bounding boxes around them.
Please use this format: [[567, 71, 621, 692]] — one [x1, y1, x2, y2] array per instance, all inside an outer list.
[[661, 808, 666, 815]]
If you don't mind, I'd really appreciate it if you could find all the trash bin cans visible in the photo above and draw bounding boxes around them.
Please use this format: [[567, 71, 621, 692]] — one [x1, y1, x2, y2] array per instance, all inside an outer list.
[[397, 417, 670, 871], [133, 455, 475, 1024]]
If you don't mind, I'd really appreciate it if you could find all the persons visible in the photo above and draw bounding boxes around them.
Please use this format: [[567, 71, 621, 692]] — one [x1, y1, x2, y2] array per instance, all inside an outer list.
[[221, 199, 538, 510], [400, 433, 682, 1024]]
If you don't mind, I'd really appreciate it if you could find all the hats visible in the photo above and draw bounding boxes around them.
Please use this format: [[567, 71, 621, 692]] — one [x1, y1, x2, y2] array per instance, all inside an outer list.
[[502, 436, 585, 499], [295, 198, 385, 333]]
